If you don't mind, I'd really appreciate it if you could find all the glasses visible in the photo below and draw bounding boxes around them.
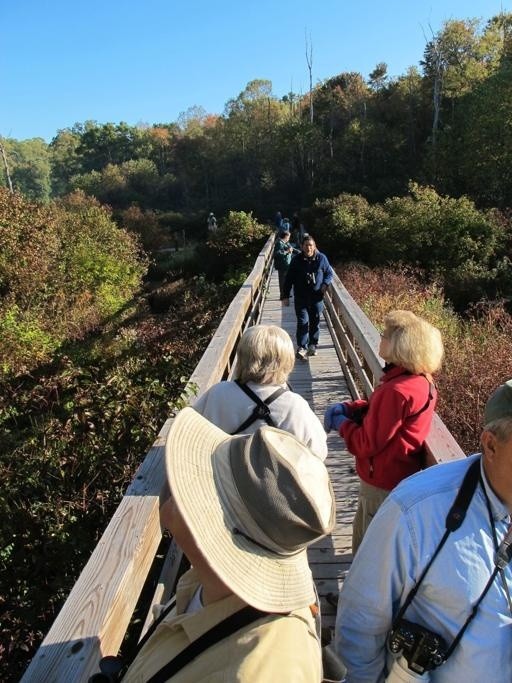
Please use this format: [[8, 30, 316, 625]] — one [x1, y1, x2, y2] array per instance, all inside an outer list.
[[379, 331, 390, 341]]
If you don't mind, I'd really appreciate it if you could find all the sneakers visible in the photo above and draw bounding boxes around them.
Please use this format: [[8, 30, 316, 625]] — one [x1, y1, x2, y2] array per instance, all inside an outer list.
[[308, 345, 318, 356], [295, 348, 308, 361]]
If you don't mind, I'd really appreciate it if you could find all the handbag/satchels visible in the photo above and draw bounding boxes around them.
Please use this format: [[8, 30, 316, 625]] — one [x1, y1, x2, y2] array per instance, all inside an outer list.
[[273, 250, 290, 264]]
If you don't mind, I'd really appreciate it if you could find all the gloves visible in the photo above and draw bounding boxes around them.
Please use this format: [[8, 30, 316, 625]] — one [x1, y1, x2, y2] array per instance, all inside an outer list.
[[330, 413, 349, 431], [323, 403, 345, 433]]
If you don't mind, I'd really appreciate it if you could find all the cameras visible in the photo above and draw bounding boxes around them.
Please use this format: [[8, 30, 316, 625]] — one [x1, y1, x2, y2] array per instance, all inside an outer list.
[[287, 245, 300, 254], [302, 270, 318, 289], [384, 622, 448, 675]]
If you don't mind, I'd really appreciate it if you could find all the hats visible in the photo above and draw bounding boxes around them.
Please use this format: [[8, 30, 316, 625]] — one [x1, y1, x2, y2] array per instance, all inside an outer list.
[[165, 406, 337, 614], [484, 380, 512, 424]]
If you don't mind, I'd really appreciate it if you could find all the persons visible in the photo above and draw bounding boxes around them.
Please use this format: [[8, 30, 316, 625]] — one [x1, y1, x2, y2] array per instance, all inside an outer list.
[[324, 310, 444, 608], [192, 324, 328, 463], [329, 378, 512, 683], [273, 212, 332, 361], [207, 212, 218, 233], [117, 406, 337, 682]]
